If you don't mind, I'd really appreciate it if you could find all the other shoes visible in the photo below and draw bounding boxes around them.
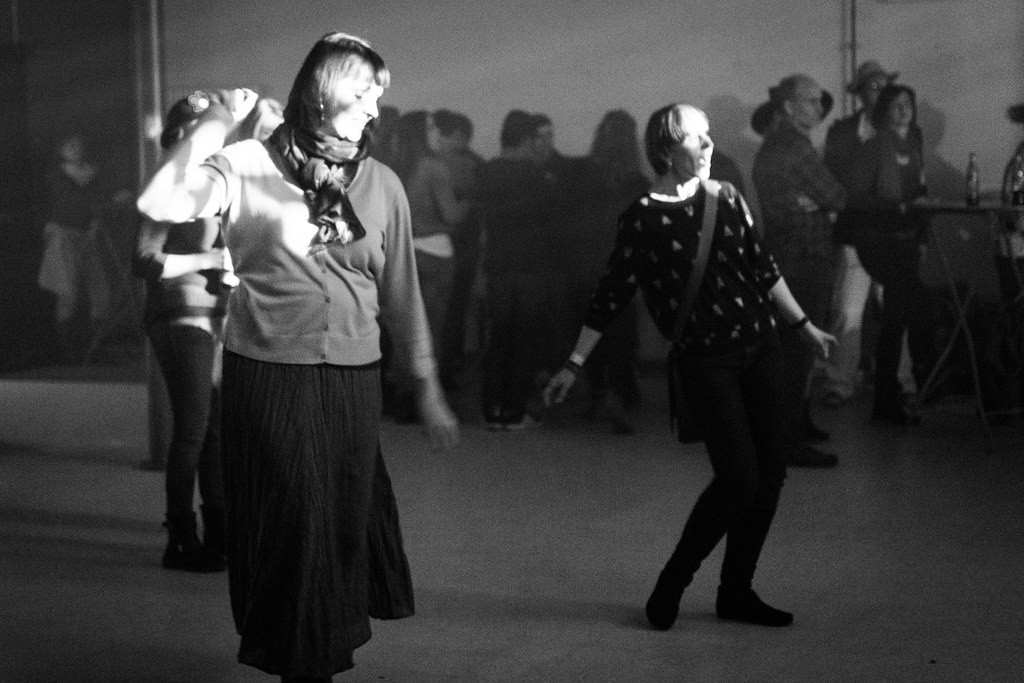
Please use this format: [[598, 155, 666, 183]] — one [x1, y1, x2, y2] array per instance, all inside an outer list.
[[789, 397, 839, 468], [162, 504, 227, 572], [484, 405, 545, 431]]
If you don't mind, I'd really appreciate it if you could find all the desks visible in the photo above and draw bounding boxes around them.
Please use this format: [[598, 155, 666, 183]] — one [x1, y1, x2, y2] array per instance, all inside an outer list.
[[86, 206, 148, 367], [897, 198, 1024, 454]]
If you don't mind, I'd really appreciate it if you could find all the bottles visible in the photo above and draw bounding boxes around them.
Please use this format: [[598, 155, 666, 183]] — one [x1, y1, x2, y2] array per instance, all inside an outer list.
[[966, 150, 979, 204]]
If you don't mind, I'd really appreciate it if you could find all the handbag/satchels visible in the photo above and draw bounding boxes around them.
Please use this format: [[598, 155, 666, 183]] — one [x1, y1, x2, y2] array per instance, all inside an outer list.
[[668, 346, 709, 444]]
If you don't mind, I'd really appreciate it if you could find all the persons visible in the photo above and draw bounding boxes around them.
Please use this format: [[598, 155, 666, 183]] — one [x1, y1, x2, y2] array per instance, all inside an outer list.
[[543, 105, 839, 629], [136, 29, 466, 683], [39, 62, 1024, 441]]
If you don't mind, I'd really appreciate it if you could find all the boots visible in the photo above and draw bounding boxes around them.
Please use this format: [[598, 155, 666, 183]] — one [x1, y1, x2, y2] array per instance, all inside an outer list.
[[716, 486, 794, 627], [646, 477, 759, 631]]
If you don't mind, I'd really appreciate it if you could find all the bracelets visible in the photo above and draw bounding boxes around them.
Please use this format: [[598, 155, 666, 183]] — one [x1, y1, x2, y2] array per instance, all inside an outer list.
[[790, 315, 810, 328], [565, 361, 582, 380], [569, 352, 586, 367]]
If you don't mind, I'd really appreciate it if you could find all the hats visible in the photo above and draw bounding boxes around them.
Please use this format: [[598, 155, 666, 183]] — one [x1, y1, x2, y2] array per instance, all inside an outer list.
[[847, 60, 902, 94], [160, 90, 210, 149]]
